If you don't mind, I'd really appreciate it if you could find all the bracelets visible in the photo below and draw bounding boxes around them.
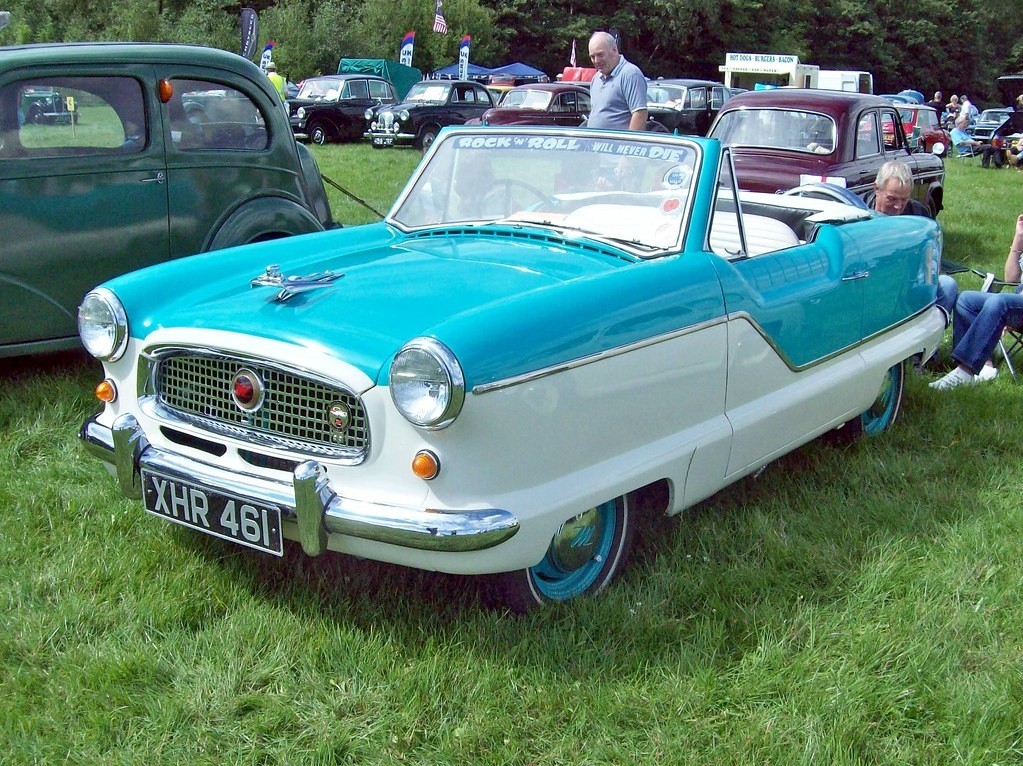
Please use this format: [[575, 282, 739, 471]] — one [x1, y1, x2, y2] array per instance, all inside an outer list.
[[1010, 247, 1022, 253]]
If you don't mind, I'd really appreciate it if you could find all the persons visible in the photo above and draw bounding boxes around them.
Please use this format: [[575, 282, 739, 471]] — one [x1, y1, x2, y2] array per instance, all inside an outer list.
[[950, 116, 1003, 169], [1006, 133, 1023, 164], [589, 32, 648, 130], [929, 214, 1023, 391], [264, 62, 288, 100], [860, 162, 958, 377], [928, 91, 971, 156]]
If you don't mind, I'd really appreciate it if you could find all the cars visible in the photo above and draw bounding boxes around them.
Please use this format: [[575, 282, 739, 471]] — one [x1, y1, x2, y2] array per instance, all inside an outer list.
[[284, 73, 400, 147], [965, 72, 1023, 169], [464, 80, 591, 130], [0, 42, 343, 361], [646, 79, 953, 218], [363, 77, 496, 154]]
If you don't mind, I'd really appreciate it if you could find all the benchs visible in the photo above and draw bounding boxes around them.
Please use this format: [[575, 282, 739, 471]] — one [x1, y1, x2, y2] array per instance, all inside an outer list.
[[172, 119, 268, 151], [560, 204, 800, 266]]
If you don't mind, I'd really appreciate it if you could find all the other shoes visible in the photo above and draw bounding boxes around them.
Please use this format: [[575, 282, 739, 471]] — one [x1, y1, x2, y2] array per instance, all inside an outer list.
[[912, 353, 950, 376]]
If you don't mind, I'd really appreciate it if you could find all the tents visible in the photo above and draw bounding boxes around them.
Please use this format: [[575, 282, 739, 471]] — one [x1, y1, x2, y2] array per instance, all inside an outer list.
[[432, 62, 546, 86], [337, 59, 423, 102]]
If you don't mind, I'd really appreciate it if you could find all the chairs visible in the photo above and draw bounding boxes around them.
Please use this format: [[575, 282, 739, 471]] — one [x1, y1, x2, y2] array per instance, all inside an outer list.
[[957, 143, 982, 170], [347, 82, 363, 96], [458, 87, 475, 101], [940, 256, 1023, 383], [668, 90, 682, 102], [987, 115, 1000, 122], [906, 126, 925, 154]]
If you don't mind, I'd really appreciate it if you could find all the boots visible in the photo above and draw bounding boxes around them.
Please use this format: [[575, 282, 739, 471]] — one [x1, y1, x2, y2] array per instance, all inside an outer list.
[[1006, 150, 1018, 165]]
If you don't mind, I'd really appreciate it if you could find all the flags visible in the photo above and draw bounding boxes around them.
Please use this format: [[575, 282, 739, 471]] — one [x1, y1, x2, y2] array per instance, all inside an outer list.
[[434, 8, 447, 34]]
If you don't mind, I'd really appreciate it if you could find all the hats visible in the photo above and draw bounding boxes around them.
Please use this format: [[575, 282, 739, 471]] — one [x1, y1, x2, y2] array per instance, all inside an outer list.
[[263, 62, 278, 70]]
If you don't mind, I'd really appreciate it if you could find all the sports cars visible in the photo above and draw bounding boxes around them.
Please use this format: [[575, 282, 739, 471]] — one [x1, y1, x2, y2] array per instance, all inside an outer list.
[[75, 125, 953, 618]]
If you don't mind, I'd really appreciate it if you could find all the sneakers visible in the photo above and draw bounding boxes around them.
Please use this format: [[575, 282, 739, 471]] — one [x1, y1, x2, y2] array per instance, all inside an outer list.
[[974, 365, 998, 382], [928, 367, 975, 391]]
